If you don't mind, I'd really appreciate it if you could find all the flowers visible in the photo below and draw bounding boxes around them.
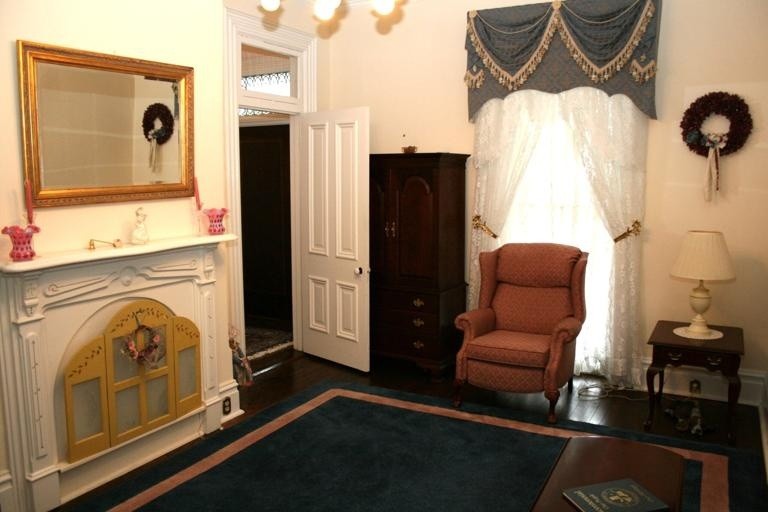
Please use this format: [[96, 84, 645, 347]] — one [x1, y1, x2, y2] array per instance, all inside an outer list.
[[123, 323, 161, 362], [230, 336, 252, 389]]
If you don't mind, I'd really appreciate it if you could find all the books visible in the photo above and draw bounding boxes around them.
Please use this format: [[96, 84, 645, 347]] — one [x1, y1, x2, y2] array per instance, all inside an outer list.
[[563, 478, 669, 512]]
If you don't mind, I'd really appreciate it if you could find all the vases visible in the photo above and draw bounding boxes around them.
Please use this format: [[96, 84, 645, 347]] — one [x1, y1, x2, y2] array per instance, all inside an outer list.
[[203, 207, 228, 235], [1, 224, 40, 263]]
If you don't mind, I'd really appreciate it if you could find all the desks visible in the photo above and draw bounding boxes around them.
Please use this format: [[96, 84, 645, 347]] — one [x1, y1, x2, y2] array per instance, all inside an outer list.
[[643, 318, 746, 443], [527, 434, 687, 512]]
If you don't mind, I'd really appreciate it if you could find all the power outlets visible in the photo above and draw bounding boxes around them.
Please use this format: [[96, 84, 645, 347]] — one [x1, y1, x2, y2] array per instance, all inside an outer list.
[[688, 378, 702, 395]]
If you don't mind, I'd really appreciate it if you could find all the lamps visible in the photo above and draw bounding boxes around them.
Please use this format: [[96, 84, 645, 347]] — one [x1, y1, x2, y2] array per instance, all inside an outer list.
[[667, 228, 738, 340]]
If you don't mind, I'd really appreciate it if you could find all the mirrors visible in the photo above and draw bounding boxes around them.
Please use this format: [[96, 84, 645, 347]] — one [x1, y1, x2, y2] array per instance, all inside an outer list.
[[15, 36, 196, 210]]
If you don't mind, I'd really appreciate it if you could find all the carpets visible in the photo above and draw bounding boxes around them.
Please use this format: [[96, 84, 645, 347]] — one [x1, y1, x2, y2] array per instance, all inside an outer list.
[[49, 379, 745, 511]]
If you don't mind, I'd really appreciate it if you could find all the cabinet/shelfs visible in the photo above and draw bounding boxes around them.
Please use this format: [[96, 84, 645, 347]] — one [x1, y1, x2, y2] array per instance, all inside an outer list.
[[369, 151, 472, 385]]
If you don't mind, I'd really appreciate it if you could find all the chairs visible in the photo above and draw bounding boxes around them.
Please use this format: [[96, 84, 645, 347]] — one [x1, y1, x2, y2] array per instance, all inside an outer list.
[[450, 242, 588, 426]]
